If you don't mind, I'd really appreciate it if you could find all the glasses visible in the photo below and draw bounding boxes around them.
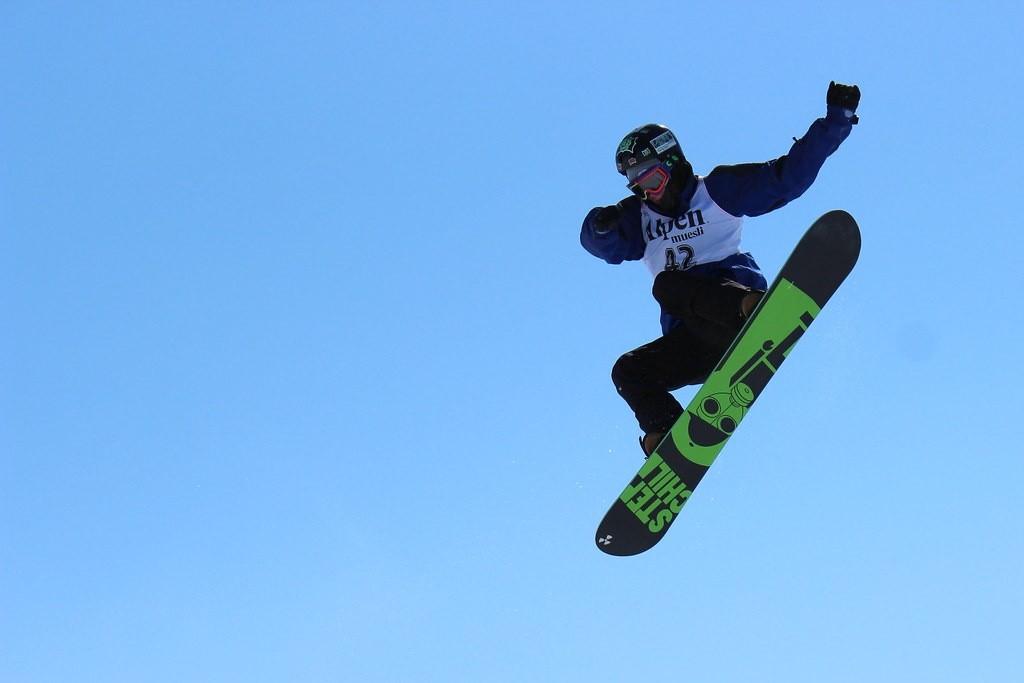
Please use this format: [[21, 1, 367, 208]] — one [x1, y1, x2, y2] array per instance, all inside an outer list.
[[627, 163, 670, 200]]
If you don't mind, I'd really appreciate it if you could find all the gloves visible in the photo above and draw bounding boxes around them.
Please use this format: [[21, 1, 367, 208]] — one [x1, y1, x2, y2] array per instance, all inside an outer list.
[[827, 81, 860, 124], [594, 206, 621, 234]]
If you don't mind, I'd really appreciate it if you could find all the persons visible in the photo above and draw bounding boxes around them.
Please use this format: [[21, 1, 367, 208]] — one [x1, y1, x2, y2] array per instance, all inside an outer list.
[[579, 81, 862, 457]]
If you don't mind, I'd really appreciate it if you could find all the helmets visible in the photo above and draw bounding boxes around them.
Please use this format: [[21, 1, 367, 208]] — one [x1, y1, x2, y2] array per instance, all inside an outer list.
[[616, 124, 692, 206]]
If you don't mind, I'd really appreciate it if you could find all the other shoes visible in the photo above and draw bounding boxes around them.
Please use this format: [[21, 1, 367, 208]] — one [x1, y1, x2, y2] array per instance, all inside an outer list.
[[741, 293, 762, 318], [639, 430, 664, 457]]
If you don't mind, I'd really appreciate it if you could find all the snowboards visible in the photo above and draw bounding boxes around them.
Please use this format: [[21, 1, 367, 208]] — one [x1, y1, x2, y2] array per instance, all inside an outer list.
[[595, 208, 862, 557]]
